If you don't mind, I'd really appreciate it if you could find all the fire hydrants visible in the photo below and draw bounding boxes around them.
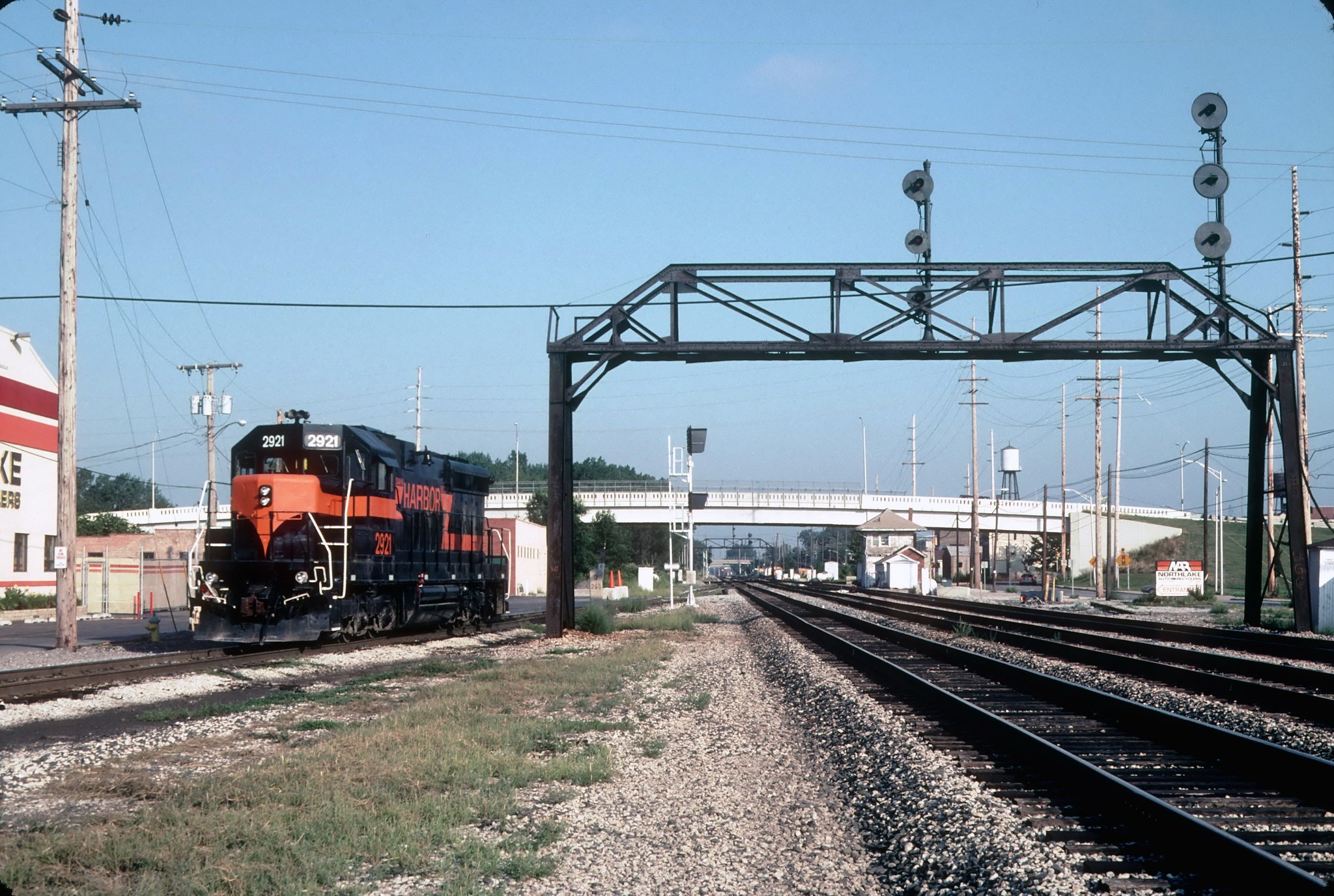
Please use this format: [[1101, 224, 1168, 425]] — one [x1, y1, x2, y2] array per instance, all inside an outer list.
[[143, 610, 164, 653]]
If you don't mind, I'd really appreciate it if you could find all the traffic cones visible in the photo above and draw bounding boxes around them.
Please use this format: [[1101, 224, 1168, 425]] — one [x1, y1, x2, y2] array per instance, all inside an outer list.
[[607, 570, 615, 588], [616, 569, 623, 587]]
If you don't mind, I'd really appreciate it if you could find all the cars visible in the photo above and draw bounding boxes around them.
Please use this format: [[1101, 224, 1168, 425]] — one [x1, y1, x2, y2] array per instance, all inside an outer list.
[[1019, 574, 1037, 586], [1140, 585, 1156, 595]]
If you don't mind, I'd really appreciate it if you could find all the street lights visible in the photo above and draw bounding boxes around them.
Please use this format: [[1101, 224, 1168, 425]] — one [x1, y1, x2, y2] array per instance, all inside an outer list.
[[1183, 458, 1224, 596], [207, 419, 248, 527], [151, 430, 161, 508], [1175, 440, 1190, 510]]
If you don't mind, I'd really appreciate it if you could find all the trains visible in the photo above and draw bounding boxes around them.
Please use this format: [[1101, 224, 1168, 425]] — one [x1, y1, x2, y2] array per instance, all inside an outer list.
[[185, 407, 513, 654], [721, 566, 734, 576]]
